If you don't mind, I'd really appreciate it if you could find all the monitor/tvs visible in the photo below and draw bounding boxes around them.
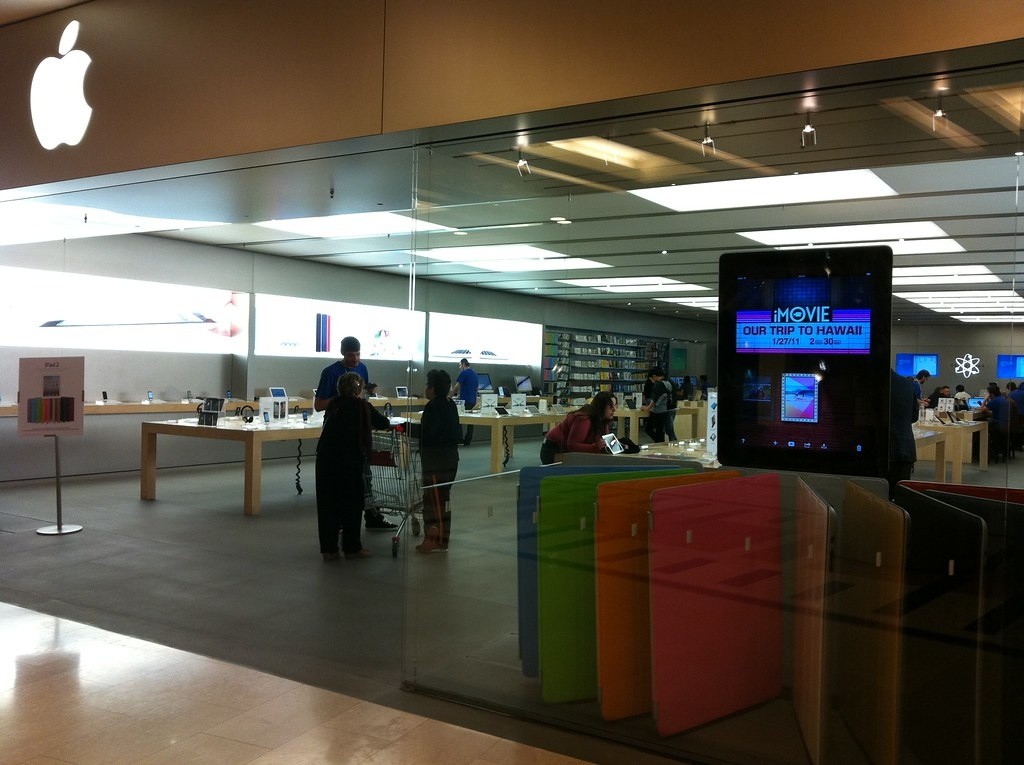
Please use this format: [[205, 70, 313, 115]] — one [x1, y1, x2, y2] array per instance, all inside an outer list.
[[896, 353, 938, 375], [997, 355, 1024, 380], [476, 373, 494, 393], [513, 375, 532, 392], [717, 244, 893, 477]]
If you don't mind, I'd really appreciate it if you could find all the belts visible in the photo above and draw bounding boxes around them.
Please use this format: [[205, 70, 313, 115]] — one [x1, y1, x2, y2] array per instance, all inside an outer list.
[[542, 438, 557, 447]]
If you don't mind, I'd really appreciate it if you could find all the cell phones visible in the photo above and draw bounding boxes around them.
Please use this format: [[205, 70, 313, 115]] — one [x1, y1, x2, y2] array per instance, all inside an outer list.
[[303, 410, 308, 421], [103, 391, 107, 399], [40, 312, 217, 327], [264, 412, 269, 422], [148, 391, 152, 399], [274, 401, 286, 419], [27, 397, 74, 425], [188, 391, 192, 399], [316, 314, 330, 353]]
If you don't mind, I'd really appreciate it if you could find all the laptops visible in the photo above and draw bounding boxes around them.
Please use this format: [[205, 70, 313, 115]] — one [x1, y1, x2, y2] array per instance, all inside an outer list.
[[396, 386, 417, 399], [369, 393, 388, 401], [269, 387, 298, 401], [968, 397, 985, 410], [625, 398, 642, 411], [514, 466, 1024, 765]]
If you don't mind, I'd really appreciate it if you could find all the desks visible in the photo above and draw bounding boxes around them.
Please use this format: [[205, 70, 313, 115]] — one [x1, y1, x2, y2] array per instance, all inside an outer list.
[[912, 429, 947, 482], [613, 401, 705, 445], [402, 411, 569, 478], [915, 421, 987, 483], [554, 439, 714, 471], [141, 416, 322, 517]]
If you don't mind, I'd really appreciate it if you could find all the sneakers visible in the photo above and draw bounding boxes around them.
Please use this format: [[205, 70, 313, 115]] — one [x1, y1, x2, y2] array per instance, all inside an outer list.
[[365, 519, 399, 531]]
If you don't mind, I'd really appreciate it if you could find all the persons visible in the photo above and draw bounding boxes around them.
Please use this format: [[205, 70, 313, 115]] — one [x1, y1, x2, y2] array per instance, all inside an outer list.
[[540, 391, 618, 465], [314, 337, 399, 531], [450, 358, 478, 446], [369, 383, 384, 397], [396, 369, 459, 553], [889, 370, 1024, 501], [639, 366, 714, 443], [316, 373, 390, 561]]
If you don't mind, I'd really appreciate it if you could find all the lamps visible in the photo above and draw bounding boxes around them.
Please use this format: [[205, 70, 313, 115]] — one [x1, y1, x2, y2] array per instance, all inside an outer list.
[[802, 113, 816, 147], [515, 145, 532, 178], [933, 97, 948, 131], [701, 123, 715, 156]]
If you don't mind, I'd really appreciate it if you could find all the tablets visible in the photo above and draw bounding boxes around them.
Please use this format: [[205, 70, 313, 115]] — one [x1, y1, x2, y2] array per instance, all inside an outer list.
[[495, 407, 508, 415], [602, 433, 625, 454], [526, 406, 540, 414], [552, 404, 566, 414]]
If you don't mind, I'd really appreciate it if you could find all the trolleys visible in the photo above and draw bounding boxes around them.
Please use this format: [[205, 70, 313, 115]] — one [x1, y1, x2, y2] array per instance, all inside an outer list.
[[359, 418, 431, 557]]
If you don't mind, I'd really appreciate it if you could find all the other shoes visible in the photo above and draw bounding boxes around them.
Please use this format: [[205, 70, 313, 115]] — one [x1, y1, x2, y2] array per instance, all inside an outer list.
[[415, 539, 448, 553], [322, 552, 343, 562], [343, 548, 372, 560]]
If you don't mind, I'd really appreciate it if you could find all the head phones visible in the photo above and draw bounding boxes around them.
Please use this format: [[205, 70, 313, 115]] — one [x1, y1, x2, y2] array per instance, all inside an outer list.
[[240, 405, 255, 423]]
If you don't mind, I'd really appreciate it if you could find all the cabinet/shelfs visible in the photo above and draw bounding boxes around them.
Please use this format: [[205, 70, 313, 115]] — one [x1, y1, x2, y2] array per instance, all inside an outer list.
[[569, 335, 667, 407]]
